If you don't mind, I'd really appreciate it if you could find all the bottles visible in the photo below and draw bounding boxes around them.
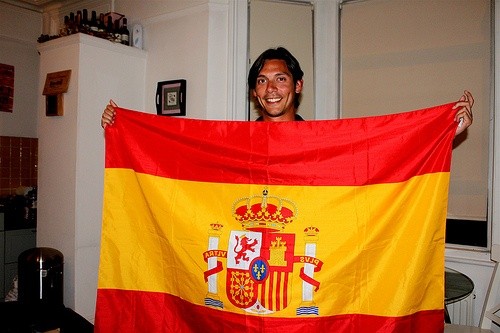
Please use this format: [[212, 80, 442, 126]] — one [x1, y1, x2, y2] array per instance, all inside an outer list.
[[113, 20, 122, 44], [106, 16, 114, 42], [121, 18, 129, 45], [97, 14, 106, 39], [89, 10, 98, 37], [62, 11, 81, 36], [81, 9, 89, 34]]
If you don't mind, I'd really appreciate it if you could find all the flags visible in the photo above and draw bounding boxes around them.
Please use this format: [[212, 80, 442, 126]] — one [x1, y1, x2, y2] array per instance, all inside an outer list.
[[94, 100, 467, 333]]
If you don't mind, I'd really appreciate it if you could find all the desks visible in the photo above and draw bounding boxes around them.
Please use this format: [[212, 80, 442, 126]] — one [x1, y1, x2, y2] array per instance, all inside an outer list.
[[445, 266, 474, 325]]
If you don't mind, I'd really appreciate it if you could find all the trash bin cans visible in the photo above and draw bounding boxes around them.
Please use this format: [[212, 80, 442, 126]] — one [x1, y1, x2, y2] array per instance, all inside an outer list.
[[18, 247, 65, 328]]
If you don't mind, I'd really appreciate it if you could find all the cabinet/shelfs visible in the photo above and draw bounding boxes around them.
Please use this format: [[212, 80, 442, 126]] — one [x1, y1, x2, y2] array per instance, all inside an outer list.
[[0, 212, 36, 302]]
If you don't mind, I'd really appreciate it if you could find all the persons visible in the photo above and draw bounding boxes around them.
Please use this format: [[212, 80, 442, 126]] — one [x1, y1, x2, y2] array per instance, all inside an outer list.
[[101, 47, 475, 135]]
